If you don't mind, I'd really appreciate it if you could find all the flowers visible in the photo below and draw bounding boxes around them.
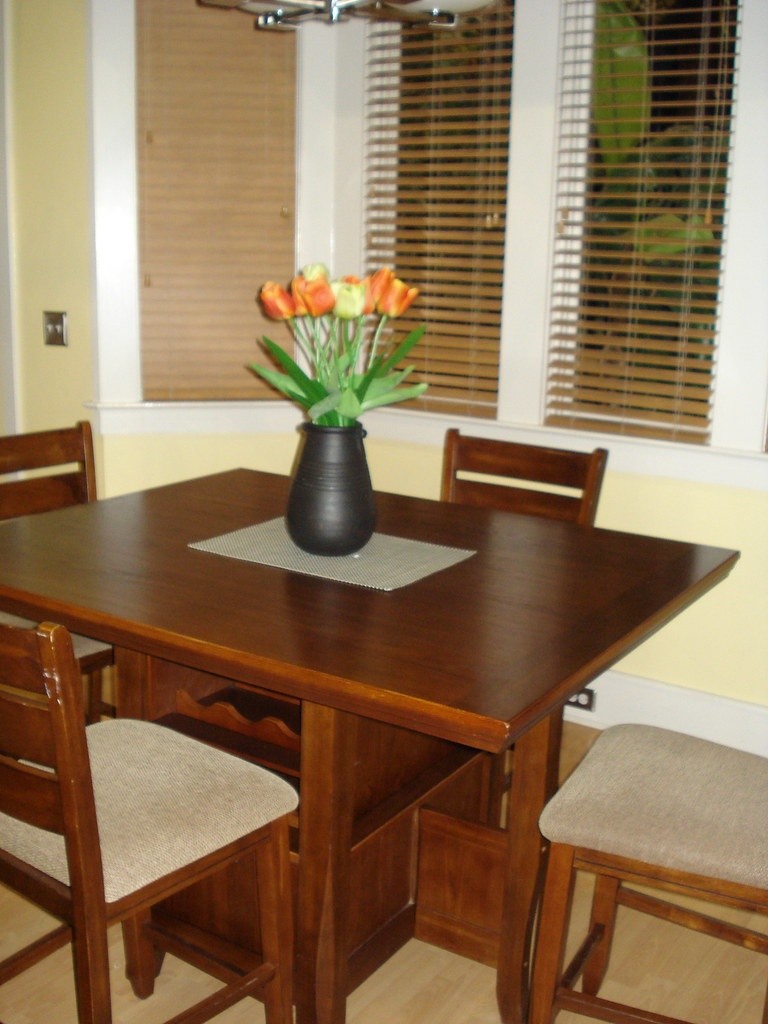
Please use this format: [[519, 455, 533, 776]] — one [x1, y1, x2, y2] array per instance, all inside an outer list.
[[245, 263, 427, 430]]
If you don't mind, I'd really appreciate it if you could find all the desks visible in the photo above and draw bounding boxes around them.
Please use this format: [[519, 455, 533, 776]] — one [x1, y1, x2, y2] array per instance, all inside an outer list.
[[0, 467, 741, 1024]]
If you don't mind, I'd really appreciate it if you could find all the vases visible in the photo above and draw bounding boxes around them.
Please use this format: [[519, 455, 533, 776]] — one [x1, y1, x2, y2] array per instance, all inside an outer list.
[[286, 421, 376, 556]]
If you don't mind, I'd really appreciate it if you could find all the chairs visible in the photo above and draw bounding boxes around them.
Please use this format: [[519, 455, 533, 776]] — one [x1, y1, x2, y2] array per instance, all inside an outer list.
[[527, 724, 768, 1024], [0, 617, 300, 1024], [439, 428, 609, 527], [0, 420, 120, 726]]
